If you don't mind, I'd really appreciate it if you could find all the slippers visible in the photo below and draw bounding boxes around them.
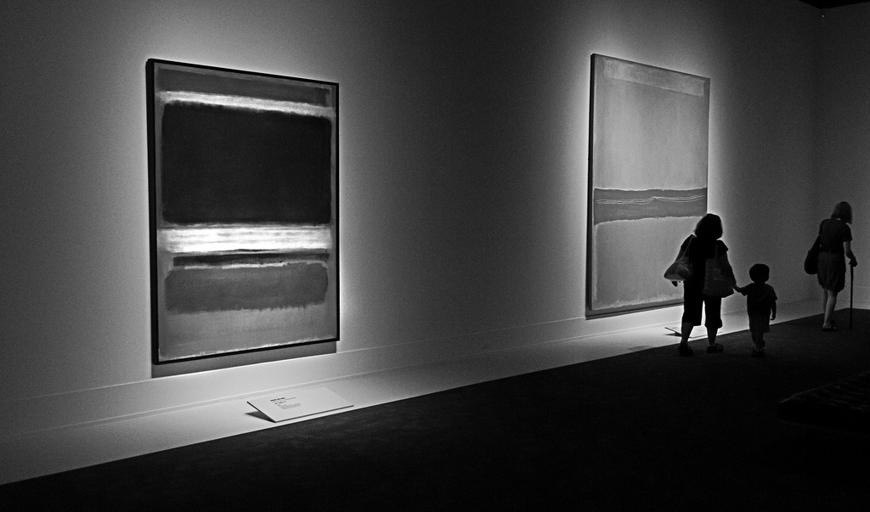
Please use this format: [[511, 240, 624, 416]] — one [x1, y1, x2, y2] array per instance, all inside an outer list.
[[821, 324, 837, 331]]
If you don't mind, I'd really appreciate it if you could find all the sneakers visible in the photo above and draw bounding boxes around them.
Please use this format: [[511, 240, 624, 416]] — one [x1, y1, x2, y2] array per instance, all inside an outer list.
[[677, 343, 695, 356], [750, 341, 768, 353], [706, 343, 724, 353]]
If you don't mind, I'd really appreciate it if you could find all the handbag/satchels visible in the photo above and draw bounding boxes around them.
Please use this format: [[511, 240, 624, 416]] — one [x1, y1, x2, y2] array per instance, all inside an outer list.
[[664, 256, 696, 282], [701, 256, 733, 298], [802, 237, 821, 275]]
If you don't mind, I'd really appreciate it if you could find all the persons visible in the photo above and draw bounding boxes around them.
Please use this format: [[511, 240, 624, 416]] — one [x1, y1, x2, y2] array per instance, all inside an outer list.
[[814, 198, 858, 332], [670, 213, 739, 357], [733, 264, 779, 351]]
